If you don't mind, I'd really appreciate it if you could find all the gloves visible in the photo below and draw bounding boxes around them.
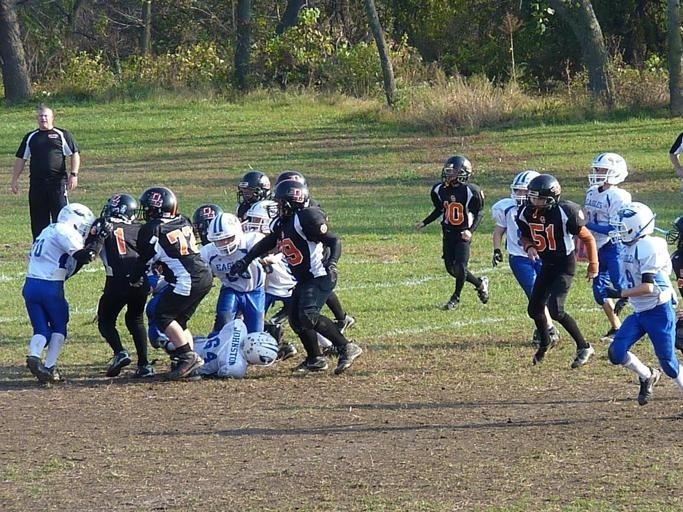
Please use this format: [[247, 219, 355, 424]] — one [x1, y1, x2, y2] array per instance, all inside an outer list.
[[96, 222, 114, 241], [231, 260, 248, 276], [492, 249, 503, 266], [599, 286, 621, 298], [323, 262, 337, 282], [613, 299, 628, 315]]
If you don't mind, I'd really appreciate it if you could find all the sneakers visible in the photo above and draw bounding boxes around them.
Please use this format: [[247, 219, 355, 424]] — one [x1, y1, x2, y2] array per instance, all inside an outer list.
[[445, 294, 459, 310], [532, 326, 561, 344], [157, 337, 205, 382], [638, 365, 660, 406], [571, 343, 595, 369], [134, 364, 155, 377], [600, 327, 620, 342], [106, 350, 131, 377], [25, 355, 66, 383], [292, 313, 362, 376], [266, 307, 297, 363], [476, 276, 489, 303], [532, 339, 557, 366]]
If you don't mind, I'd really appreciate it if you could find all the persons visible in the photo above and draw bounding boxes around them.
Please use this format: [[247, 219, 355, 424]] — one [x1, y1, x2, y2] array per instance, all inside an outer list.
[[412, 155, 489, 310], [669, 132, 683, 178], [583, 152, 632, 339], [10, 105, 80, 243], [490, 169, 563, 348], [20, 168, 366, 385], [665, 214, 683, 353], [600, 201, 683, 406], [514, 172, 600, 368]]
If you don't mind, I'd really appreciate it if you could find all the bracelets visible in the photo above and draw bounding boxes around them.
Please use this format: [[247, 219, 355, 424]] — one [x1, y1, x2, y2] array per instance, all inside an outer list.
[[70, 172, 78, 177]]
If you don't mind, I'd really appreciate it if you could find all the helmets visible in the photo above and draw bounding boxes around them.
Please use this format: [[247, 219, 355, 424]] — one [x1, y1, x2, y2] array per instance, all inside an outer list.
[[242, 332, 278, 366], [608, 202, 655, 243], [587, 152, 628, 188], [101, 193, 138, 221], [191, 204, 242, 257], [134, 187, 178, 221], [510, 170, 562, 216], [57, 203, 95, 239], [237, 172, 271, 204], [442, 156, 471, 190], [666, 216, 683, 252], [272, 171, 310, 218]]
